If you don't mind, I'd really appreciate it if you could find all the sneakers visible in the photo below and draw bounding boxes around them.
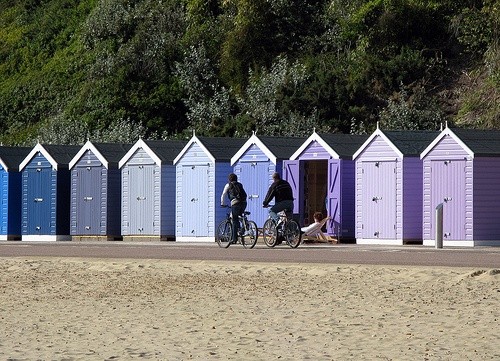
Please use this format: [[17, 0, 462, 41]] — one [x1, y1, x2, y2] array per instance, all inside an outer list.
[[275, 218, 284, 229]]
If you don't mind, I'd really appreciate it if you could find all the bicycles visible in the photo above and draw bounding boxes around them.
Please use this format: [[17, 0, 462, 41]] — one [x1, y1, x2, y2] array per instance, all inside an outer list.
[[216, 204, 259, 250], [263, 205, 302, 249]]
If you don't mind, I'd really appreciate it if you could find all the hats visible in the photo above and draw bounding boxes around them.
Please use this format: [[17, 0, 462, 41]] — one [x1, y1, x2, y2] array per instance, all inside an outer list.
[[273, 172, 280, 178]]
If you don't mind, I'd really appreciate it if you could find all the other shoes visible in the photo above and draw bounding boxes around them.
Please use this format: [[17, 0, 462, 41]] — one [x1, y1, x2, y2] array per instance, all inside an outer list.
[[229, 239, 238, 243]]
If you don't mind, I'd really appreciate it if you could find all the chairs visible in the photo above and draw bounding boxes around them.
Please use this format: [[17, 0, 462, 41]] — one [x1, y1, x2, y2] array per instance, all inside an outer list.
[[290, 217, 333, 245]]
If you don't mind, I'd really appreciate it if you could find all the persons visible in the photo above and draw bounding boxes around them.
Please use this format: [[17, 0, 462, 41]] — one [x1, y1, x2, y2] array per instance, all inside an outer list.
[[263, 172, 294, 236], [221, 173, 247, 244], [301, 212, 332, 237]]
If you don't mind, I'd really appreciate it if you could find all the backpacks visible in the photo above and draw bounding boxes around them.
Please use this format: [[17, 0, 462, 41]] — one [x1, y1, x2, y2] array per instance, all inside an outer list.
[[229, 181, 247, 202]]
